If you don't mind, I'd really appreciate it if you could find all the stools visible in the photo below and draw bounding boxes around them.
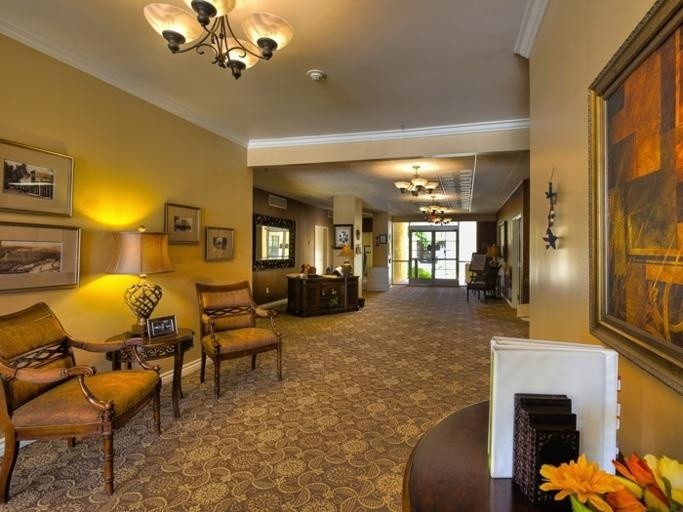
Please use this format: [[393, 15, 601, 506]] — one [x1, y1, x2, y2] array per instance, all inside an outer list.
[[466, 273, 492, 301]]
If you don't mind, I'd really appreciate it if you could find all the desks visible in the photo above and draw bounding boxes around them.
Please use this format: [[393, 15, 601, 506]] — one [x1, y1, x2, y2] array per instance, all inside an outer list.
[[401, 399, 683, 511], [104, 327, 194, 419], [495, 275, 501, 295], [285, 272, 359, 318], [366, 267, 388, 292]]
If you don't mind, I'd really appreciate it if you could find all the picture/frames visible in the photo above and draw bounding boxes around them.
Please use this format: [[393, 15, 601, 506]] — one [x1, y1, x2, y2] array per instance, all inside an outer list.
[[204, 227, 234, 263], [586, 0, 682, 395], [379, 234, 387, 244], [497, 220, 507, 258], [0, 137, 73, 218], [147, 315, 178, 342], [0, 222, 81, 294], [163, 202, 201, 245], [332, 224, 353, 250]]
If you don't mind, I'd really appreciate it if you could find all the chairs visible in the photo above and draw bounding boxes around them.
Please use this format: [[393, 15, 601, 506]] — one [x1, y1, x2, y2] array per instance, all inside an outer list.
[[0, 300, 162, 504], [194, 281, 283, 399], [466, 266, 501, 304]]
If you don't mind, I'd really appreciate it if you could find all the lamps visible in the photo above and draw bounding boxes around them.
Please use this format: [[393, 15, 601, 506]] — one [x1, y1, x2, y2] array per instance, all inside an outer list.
[[103, 225, 174, 339], [140, 1, 296, 82], [393, 166, 440, 196], [485, 243, 501, 265], [417, 196, 448, 214], [431, 212, 450, 226], [336, 244, 355, 277]]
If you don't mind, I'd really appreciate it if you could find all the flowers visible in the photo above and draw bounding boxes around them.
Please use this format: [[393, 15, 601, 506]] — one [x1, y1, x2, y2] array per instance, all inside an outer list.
[[539, 445, 682, 512]]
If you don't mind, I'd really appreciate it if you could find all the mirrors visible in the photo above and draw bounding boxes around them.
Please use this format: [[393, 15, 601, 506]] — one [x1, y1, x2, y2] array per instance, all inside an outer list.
[[253, 212, 296, 272]]
